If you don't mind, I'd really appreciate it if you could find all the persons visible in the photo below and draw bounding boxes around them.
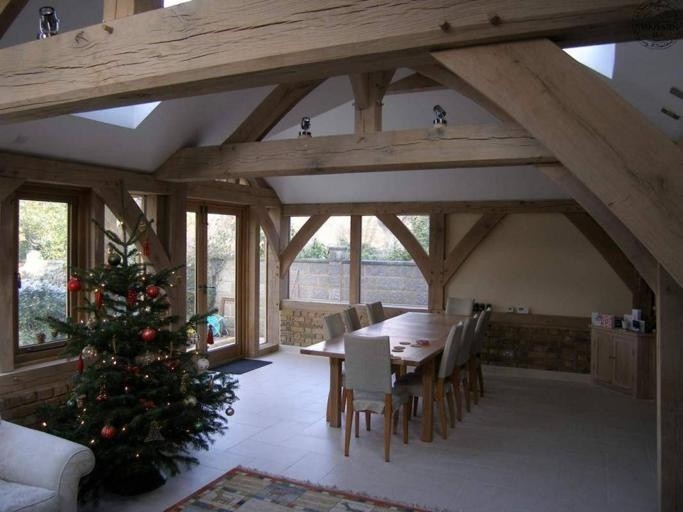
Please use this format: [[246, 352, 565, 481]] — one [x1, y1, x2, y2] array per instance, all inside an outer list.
[[21, 239, 45, 278]]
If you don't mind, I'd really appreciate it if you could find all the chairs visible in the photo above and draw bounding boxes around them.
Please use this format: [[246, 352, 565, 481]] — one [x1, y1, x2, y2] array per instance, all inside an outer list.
[[322, 298, 493, 463]]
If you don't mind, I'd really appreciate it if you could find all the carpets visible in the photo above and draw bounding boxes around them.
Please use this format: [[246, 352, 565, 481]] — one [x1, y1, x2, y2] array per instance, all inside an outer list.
[[161, 463, 455, 512], [209, 357, 273, 375]]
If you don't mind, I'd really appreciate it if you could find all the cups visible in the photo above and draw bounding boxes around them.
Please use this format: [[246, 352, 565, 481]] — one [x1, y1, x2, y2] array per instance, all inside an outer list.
[[622, 319, 630, 330]]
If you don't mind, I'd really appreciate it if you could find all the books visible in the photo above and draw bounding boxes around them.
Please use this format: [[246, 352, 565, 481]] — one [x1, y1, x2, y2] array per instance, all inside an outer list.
[[591, 307, 645, 332]]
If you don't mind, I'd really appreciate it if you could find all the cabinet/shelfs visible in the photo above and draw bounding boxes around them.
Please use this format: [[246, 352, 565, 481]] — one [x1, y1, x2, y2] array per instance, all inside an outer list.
[[588, 326, 656, 399]]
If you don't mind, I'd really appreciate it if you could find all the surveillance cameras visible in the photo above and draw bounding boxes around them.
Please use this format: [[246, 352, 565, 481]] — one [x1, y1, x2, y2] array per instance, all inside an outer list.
[[299, 115, 312, 131], [433, 102, 447, 120], [39, 6, 60, 36]]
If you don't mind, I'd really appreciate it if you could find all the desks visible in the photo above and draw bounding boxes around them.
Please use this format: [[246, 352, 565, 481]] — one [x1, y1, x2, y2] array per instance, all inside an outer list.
[[300, 312, 479, 442]]
